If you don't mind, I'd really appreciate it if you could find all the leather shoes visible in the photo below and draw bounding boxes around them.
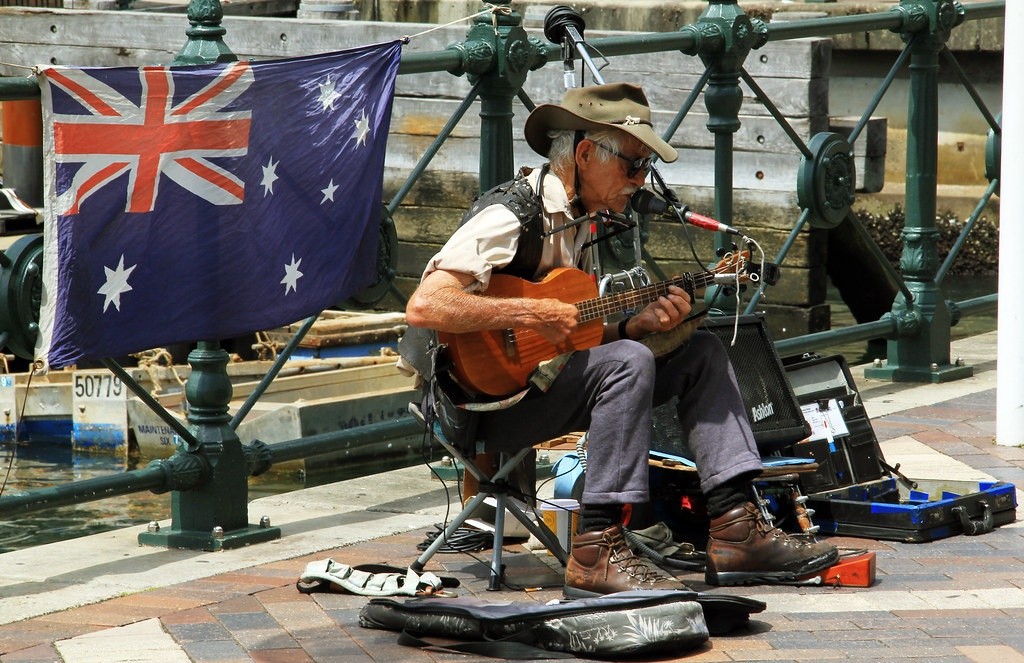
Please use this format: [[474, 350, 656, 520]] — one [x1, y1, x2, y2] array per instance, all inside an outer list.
[[563, 524, 696, 601], [706, 503, 840, 586]]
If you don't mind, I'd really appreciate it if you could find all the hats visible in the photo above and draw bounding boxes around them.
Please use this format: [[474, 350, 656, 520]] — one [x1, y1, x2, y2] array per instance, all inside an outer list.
[[523, 83, 679, 163]]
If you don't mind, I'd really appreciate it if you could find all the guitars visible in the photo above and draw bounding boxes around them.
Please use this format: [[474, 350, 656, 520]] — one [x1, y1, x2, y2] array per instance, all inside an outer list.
[[438, 250, 751, 399]]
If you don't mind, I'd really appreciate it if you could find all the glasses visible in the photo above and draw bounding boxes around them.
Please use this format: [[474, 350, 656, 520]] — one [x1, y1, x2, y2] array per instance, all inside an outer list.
[[594, 142, 653, 178]]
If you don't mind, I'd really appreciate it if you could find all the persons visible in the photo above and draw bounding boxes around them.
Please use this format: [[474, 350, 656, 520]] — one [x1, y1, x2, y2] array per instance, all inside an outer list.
[[406, 83, 839, 602]]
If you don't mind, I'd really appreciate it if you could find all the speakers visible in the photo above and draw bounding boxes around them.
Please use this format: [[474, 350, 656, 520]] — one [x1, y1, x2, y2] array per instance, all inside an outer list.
[[646, 311, 812, 460]]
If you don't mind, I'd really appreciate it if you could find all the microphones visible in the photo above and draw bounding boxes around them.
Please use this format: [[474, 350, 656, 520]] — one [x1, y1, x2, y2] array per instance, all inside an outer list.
[[630, 189, 740, 236]]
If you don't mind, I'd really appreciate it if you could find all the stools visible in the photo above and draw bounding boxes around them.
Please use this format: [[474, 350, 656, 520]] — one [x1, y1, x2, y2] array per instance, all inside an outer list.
[[405, 398, 571, 598]]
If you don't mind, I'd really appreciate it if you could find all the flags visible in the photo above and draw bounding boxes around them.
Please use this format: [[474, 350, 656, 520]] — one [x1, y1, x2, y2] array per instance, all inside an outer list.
[[40, 43, 408, 366]]
